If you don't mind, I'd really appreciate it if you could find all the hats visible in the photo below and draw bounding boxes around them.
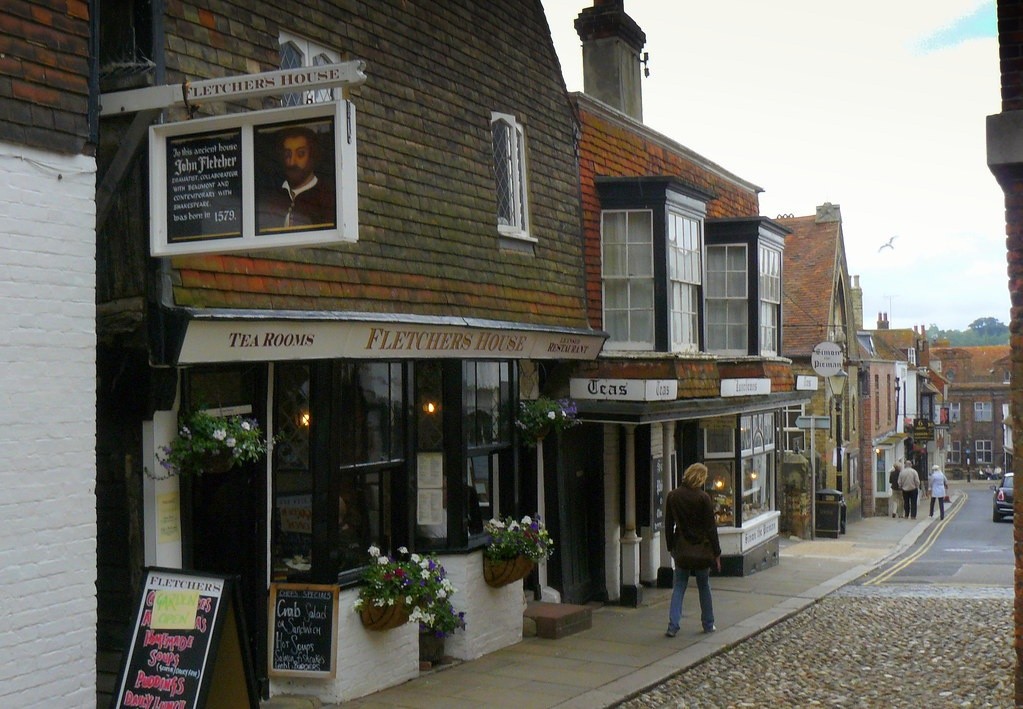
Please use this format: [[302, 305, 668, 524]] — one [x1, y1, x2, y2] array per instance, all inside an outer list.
[[932, 465, 940, 470]]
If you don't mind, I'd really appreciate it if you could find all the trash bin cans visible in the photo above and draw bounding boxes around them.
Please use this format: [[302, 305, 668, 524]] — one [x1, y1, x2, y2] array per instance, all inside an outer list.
[[953, 468, 963, 480], [815, 488, 843, 539]]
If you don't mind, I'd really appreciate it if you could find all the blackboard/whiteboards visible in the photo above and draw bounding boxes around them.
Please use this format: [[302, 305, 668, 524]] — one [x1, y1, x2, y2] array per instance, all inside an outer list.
[[111, 565, 238, 709], [649, 449, 678, 533], [266, 583, 341, 679]]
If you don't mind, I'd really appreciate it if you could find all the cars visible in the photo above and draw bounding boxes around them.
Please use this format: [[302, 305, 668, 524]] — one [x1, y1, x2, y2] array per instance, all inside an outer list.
[[989, 472, 1014, 522]]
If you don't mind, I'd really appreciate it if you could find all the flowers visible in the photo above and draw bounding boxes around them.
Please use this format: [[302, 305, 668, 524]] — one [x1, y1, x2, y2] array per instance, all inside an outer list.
[[488, 513, 554, 565], [145, 419, 276, 480], [351, 545, 466, 636], [515, 398, 582, 430]]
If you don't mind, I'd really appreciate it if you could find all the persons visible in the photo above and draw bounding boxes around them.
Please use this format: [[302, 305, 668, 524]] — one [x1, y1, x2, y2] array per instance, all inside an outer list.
[[978, 465, 1001, 480], [926, 465, 948, 520], [665, 462, 723, 636], [897, 459, 920, 520], [890, 462, 904, 518]]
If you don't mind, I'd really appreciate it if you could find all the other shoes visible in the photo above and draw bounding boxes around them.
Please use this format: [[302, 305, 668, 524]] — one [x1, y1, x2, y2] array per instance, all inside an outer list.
[[905, 516, 916, 519], [892, 513, 903, 518], [666, 631, 674, 637], [704, 625, 716, 632]]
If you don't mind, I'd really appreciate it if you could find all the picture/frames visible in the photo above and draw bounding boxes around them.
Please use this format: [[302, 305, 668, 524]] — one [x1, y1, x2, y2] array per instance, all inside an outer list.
[[267, 583, 340, 679]]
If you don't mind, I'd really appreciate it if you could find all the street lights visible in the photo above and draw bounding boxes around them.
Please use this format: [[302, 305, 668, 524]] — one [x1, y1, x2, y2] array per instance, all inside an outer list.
[[965, 435, 972, 483], [828, 367, 848, 535]]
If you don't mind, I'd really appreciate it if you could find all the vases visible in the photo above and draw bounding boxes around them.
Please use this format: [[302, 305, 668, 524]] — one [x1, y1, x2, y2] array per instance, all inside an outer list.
[[360, 596, 417, 632], [483, 554, 534, 588], [201, 447, 236, 474]]
